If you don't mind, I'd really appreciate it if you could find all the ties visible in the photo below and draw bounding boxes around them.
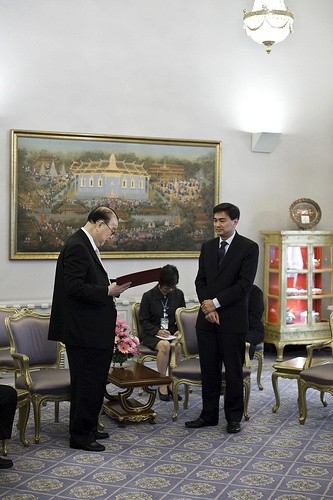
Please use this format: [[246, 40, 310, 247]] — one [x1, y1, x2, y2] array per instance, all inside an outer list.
[[217, 241, 228, 271]]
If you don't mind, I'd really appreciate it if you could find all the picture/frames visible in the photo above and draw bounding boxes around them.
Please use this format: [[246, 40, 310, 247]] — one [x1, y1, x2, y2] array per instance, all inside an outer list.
[[9, 129, 222, 260]]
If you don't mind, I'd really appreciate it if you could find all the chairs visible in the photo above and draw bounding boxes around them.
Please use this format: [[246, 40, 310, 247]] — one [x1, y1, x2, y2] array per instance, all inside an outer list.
[[131, 302, 264, 421], [0, 307, 104, 456], [299, 312, 333, 425]]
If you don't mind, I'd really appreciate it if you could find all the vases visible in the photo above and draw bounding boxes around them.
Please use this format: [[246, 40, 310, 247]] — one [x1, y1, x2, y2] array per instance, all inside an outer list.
[[112, 352, 128, 368]]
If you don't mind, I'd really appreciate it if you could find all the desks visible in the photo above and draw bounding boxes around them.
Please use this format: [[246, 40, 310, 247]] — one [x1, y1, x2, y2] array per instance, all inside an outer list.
[[271, 357, 328, 417], [101, 361, 172, 428]]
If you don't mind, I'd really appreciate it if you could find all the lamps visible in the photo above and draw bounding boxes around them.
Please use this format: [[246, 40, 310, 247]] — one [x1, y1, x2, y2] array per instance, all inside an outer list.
[[242, 0, 294, 54], [251, 131, 282, 154]]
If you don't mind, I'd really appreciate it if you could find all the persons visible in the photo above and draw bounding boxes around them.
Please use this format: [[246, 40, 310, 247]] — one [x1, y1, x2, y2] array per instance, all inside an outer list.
[[185, 203, 259, 432], [48, 206, 132, 451], [0, 385, 18, 469], [138, 264, 186, 400]]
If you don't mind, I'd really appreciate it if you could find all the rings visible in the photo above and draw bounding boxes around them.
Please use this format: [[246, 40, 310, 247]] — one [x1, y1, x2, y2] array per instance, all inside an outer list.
[[204, 310, 205, 311]]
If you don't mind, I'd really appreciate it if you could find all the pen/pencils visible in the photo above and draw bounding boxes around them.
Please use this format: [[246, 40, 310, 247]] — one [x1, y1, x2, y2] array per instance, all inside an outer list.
[[161, 325, 165, 330]]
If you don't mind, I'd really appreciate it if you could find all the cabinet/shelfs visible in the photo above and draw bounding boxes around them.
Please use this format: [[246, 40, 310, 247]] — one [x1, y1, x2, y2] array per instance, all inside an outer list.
[[261, 231, 333, 362]]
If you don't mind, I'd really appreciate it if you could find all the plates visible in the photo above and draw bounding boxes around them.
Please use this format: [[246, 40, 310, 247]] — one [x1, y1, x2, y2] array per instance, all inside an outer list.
[[289, 198, 322, 229]]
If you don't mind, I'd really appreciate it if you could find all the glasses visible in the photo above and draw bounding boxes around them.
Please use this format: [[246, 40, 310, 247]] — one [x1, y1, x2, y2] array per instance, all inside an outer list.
[[97, 220, 116, 236]]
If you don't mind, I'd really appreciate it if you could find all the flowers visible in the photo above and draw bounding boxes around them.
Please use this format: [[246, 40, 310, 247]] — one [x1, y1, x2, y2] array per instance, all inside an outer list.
[[113, 319, 141, 367]]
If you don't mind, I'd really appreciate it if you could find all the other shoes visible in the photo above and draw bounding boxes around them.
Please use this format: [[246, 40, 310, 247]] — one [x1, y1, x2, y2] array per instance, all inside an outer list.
[[157, 385, 170, 401], [0, 457, 13, 469], [168, 386, 182, 401], [220, 386, 225, 395]]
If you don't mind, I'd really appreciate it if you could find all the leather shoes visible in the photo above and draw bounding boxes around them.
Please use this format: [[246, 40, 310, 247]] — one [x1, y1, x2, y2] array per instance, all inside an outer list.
[[227, 420, 241, 434], [185, 418, 218, 428], [95, 431, 109, 440], [69, 439, 105, 452]]
